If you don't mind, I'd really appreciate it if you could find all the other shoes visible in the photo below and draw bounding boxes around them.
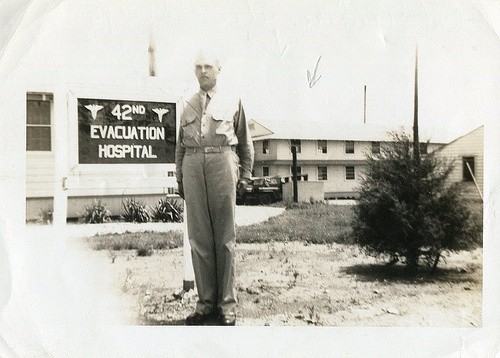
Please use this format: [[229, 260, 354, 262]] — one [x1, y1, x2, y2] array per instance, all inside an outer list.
[[185, 308, 237, 326]]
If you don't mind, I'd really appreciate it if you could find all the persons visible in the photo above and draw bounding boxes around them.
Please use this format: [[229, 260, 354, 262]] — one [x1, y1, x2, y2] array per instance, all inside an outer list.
[[170, 50, 257, 327]]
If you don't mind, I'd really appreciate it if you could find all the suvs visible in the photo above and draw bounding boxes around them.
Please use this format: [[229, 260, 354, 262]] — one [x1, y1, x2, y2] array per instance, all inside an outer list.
[[237, 176, 281, 206]]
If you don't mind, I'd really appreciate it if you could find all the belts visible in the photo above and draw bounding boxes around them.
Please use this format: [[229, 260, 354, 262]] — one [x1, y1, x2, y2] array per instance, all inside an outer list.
[[185, 146, 232, 153]]
